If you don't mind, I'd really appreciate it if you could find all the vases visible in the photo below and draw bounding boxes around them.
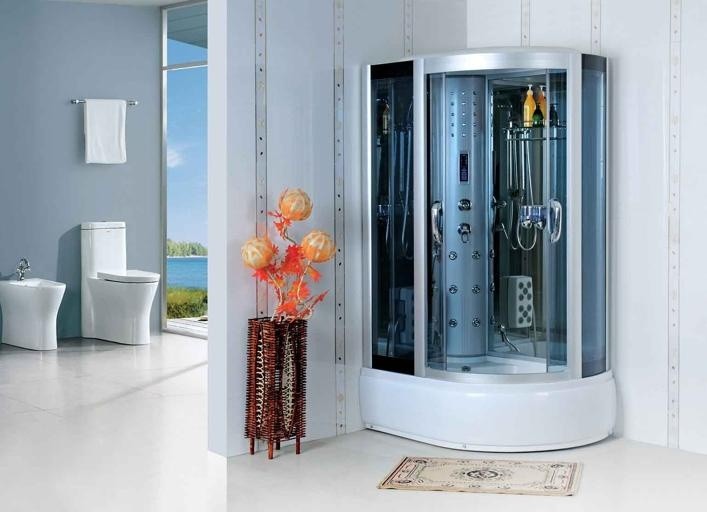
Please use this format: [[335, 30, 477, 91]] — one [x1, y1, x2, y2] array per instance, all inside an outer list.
[[244, 317, 308, 461]]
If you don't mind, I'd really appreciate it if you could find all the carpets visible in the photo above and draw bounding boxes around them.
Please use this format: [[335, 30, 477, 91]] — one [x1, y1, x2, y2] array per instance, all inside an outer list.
[[376, 454, 579, 497]]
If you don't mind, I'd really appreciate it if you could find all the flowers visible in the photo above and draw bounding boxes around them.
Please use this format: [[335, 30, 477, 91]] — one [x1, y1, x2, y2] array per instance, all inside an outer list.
[[238, 186, 336, 322]]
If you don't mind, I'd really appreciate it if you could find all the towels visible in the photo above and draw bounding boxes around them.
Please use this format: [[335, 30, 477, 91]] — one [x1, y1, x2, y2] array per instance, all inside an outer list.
[[84, 97, 126, 166]]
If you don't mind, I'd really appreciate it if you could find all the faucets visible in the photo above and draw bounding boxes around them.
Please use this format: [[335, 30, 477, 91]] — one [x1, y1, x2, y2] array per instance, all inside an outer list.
[[13, 256, 32, 280]]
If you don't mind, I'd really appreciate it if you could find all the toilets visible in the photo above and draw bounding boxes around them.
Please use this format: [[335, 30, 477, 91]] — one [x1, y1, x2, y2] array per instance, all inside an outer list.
[[79, 221, 161, 346]]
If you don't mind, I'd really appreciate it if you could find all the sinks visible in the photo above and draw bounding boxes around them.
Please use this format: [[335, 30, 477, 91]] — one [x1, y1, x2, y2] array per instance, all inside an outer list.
[[1, 278, 66, 350]]
